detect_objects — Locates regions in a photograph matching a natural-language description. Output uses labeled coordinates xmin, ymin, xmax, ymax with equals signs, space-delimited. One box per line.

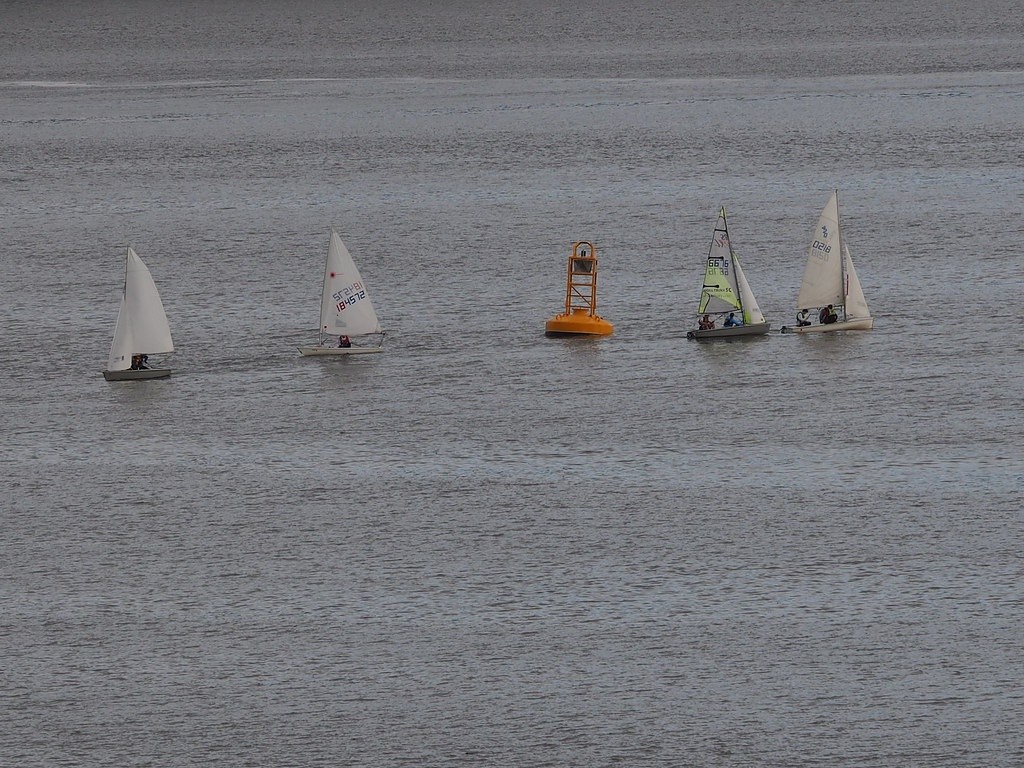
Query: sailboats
xmin=102 ymin=245 xmax=175 ymax=381
xmin=296 ymin=227 xmax=387 ymax=356
xmin=687 ymin=205 xmax=771 ymax=338
xmin=780 ymin=189 xmax=874 ymax=333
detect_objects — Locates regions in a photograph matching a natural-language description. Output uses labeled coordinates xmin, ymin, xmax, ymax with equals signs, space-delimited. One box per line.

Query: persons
xmin=796 ymin=309 xmax=811 ymax=327
xmin=339 ymin=335 xmax=351 ymax=348
xmin=132 ymin=354 xmax=149 ymax=370
xmin=824 ymin=304 xmax=838 ymax=324
xmin=724 ymin=313 xmax=740 ymax=327
xmin=698 ymin=316 xmax=714 ymax=330
